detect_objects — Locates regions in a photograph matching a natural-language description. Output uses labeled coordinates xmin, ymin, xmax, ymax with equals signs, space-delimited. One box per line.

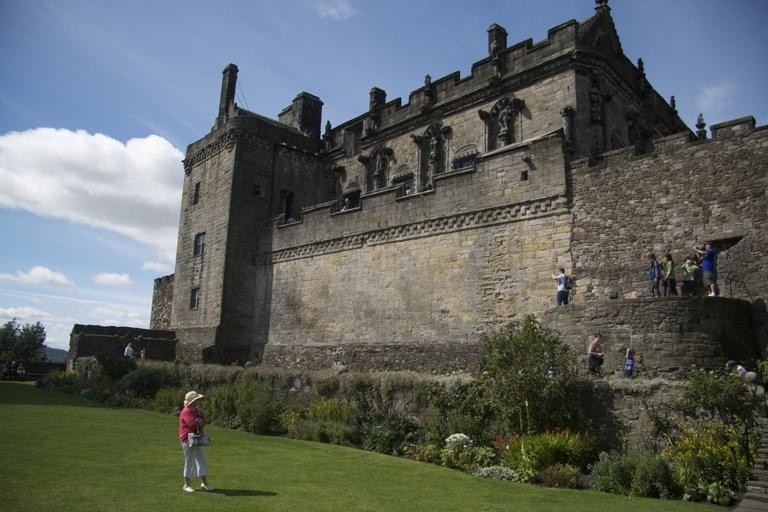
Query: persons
xmin=341 ymin=199 xmax=353 ymax=211
xmin=625 ymin=348 xmax=636 ymax=378
xmin=420 ymin=181 xmax=427 ymax=192
xmin=587 ymin=333 xmax=604 ymax=375
xmin=427 ymin=183 xmax=432 ymax=191
xmin=0 ymin=359 xmax=27 ymax=381
xmin=718 ymin=360 xmax=751 ymax=383
xmin=139 ymin=345 xmax=146 ymax=359
xmin=404 ymin=183 xmax=412 ymax=196
xmin=646 ymin=242 xmax=716 ymax=298
xmin=552 ymin=266 xmax=569 ymax=305
xmin=285 ymin=212 xmax=296 ymax=224
xmin=123 ymin=342 xmax=133 ymax=359
xmin=179 ymin=390 xmax=213 ymax=492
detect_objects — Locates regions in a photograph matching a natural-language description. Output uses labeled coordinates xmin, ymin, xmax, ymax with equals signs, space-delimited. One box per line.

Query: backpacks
xmin=563 ymin=273 xmax=573 ymax=290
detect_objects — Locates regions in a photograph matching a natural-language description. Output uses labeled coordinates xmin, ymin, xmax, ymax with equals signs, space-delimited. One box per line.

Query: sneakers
xmin=182 ymin=484 xmax=195 ymax=493
xmin=201 ymin=483 xmax=210 ymax=492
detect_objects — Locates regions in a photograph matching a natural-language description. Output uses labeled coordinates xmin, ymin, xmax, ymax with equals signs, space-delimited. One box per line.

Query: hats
xmin=184 ymin=390 xmax=204 ymax=408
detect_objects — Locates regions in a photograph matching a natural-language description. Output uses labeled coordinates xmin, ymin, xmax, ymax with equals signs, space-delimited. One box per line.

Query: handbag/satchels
xmin=188 ymin=433 xmax=210 ymax=448
